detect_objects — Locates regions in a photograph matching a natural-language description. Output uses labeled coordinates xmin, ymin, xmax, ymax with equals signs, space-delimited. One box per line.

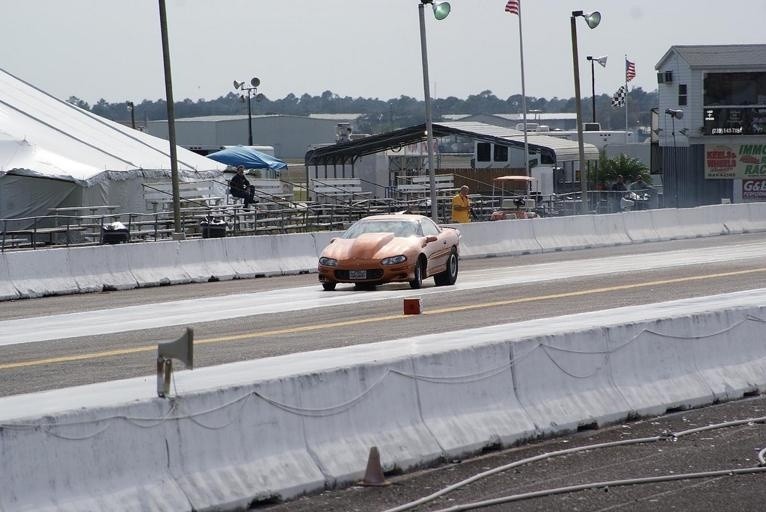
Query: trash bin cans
xmin=200 ymin=220 xmax=227 ymax=238
xmin=630 ymin=196 xmax=650 ymax=211
xmin=102 ymin=226 xmax=130 ymax=245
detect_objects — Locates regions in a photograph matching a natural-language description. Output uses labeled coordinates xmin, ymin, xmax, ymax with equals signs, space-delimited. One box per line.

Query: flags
xmin=505 ymin=0 xmax=519 ymax=14
xmin=626 ymin=60 xmax=635 ymax=82
xmin=611 ymin=85 xmax=628 ymax=107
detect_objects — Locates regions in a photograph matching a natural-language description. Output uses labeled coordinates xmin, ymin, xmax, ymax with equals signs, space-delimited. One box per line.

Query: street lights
xmin=234 ymin=76 xmax=265 ymax=145
xmin=569 ymin=8 xmax=603 ymax=212
xmin=586 ymin=55 xmax=613 ymax=124
xmin=125 ymin=101 xmax=137 ymax=128
xmin=416 ymin=0 xmax=453 ymax=223
xmin=664 ymin=107 xmax=685 ymax=205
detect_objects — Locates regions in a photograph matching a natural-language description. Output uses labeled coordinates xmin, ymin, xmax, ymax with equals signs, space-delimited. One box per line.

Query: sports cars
xmin=314 ymin=210 xmax=463 ymax=291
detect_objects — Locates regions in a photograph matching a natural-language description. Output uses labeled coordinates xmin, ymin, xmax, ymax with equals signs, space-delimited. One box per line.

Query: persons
xmin=230 ymin=165 xmax=259 ymax=212
xmin=630 ymin=175 xmax=652 ymax=190
xmin=612 ymin=175 xmax=628 ymax=190
xmin=451 ymin=185 xmax=472 ymax=223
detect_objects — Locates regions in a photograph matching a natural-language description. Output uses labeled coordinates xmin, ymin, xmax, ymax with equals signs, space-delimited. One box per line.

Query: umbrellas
xmin=206 ymin=144 xmax=288 ymax=171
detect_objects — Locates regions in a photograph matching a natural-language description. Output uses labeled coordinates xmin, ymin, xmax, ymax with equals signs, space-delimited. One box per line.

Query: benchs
xmin=0 ymin=171 xmax=542 ymax=250
